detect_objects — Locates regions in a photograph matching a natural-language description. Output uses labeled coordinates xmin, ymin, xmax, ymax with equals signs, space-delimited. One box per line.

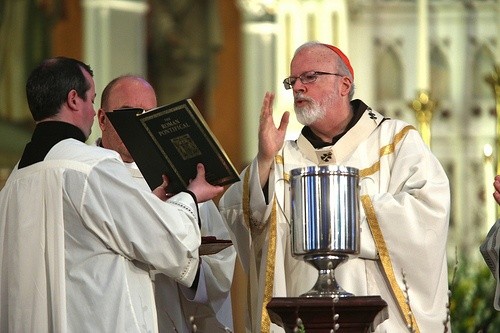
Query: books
xmin=199 ymin=237 xmax=232 ymax=255
xmin=105 ymin=99 xmax=241 ymax=198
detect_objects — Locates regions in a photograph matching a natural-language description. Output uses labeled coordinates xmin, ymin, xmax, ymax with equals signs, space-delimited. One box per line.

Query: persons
xmin=218 ymin=41 xmax=449 ymax=333
xmin=0 ymin=55 xmax=225 ymax=333
xmin=91 ymin=75 xmax=237 ymax=333
xmin=478 ymin=174 xmax=500 ymax=332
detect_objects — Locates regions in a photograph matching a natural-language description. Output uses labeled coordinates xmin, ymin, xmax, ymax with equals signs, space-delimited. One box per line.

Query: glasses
xmin=283 ymin=69 xmax=344 ymax=90
